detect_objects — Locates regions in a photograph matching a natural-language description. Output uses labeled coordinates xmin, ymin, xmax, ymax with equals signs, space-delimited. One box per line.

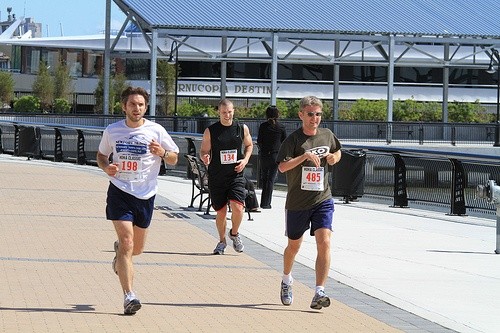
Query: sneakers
xmin=280 ymin=279 xmax=293 ymax=305
xmin=113 ymin=241 xmax=119 ymax=274
xmin=124 ymin=290 xmax=141 ymax=314
xmin=310 ymin=290 xmax=330 ymax=310
xmin=228 ymin=229 xmax=244 ymax=252
xmin=214 ymin=241 xmax=226 ymax=254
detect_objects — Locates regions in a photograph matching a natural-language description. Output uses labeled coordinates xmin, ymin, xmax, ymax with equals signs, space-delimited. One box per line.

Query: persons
xmin=200 ymin=99 xmax=253 ymax=255
xmin=276 ymin=96 xmax=342 ymax=310
xmin=258 ymin=105 xmax=287 ymax=209
xmin=98 ymin=86 xmax=180 ymax=315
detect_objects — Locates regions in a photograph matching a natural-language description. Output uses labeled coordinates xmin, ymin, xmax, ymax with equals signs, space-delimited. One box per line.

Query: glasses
xmin=303 ymin=112 xmax=322 ymax=117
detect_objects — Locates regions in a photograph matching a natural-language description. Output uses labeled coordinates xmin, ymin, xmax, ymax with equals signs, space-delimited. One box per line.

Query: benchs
xmin=183 ymin=154 xmax=254 ymax=220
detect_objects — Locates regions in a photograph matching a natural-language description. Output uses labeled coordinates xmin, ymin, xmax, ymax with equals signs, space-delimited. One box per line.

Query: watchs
xmin=159 ymin=149 xmax=168 ymax=159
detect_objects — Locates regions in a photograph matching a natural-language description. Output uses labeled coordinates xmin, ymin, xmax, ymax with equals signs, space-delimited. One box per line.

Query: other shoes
xmin=245 ymin=208 xmax=260 ymax=212
